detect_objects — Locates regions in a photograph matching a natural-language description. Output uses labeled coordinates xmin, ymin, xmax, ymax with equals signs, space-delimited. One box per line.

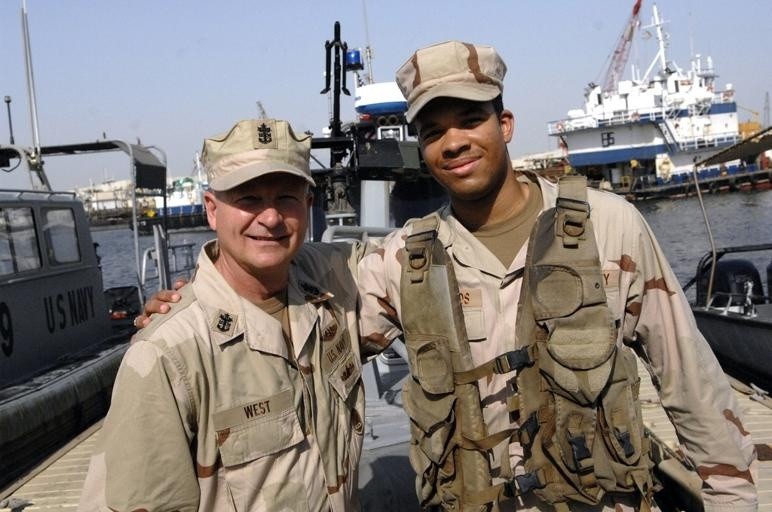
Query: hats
xmin=395 ymin=41 xmax=507 ymax=125
xmin=199 ymin=118 xmax=317 ymax=192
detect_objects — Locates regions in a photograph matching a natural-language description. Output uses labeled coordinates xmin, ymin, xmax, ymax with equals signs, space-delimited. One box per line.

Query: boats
xmin=0 ymin=2 xmax=195 ymax=471
xmin=1 ymin=178 xmax=134 ymax=238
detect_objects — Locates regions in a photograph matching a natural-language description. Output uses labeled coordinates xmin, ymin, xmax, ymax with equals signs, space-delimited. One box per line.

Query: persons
xmin=76 ymin=120 xmax=382 ymax=511
xmin=133 ymin=41 xmax=759 ymax=511
